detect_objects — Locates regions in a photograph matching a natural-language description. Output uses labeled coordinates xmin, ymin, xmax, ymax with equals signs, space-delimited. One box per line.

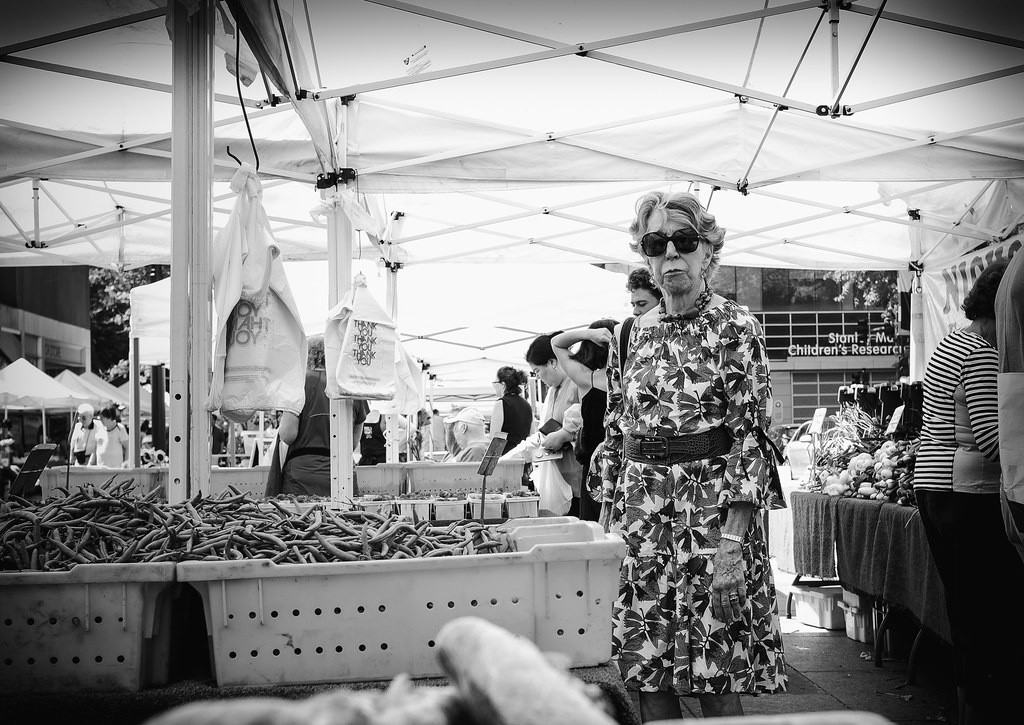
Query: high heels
xmin=956 ymin=675 xmax=999 ymax=724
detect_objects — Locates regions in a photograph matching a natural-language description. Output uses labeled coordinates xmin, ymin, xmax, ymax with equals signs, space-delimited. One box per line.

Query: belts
xmin=624 ymin=425 xmax=731 ymax=466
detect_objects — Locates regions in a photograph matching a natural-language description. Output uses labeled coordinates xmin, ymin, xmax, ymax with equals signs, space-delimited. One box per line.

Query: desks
xmin=786 ymin=492 xmax=951 ymax=689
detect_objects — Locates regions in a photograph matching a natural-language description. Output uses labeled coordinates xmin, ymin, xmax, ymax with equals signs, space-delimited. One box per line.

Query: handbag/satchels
xmin=264 ymin=433 xmax=282 ymax=497
xmin=74 ymin=450 xmax=86 ymax=465
xmin=997 ymin=372 xmax=1024 ymax=504
xmin=530 ymin=459 xmax=573 ymax=516
xmin=573 ymin=427 xmax=588 ymax=466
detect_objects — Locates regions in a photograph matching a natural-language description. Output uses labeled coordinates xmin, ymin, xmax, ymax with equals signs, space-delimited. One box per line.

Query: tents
xmin=129 ymin=266 xmax=633 ymax=468
xmin=2 ymin=0 xmax=1024 ymax=506
xmin=0 ymin=358 xmax=169 ymax=444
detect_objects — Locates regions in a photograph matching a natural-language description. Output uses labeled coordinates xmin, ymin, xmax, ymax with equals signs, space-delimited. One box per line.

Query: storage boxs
xmin=1 ymin=455 xmax=627 ymax=705
xmin=836 ymin=585 xmax=873 ymax=644
xmin=871 ymin=597 xmax=931 ymax=660
xmin=790 ymin=585 xmax=846 ymax=630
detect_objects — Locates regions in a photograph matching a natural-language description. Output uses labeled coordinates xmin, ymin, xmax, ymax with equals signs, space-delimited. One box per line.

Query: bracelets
xmin=721 ymin=533 xmax=744 ymax=551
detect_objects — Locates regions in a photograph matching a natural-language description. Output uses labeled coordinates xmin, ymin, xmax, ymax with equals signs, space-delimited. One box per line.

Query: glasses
xmin=640 ymin=227 xmax=710 ymax=257
xmin=492 ymin=381 xmax=503 ymax=387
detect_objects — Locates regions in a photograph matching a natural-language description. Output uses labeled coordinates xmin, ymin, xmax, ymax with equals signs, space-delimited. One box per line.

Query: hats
xmin=443 ymin=407 xmax=486 ymax=426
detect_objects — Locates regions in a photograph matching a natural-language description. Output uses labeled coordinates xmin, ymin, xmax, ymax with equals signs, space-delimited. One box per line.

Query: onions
xmin=818 ymin=440 xmax=897 ymax=501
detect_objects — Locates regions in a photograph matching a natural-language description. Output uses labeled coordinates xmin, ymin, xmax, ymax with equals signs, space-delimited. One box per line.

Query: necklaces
xmin=659 ymin=278 xmax=714 ymax=322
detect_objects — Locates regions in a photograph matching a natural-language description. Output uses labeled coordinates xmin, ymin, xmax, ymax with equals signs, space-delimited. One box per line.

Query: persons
xmin=212 ymin=414 xmax=225 ymax=454
xmin=433 ymin=409 xmax=445 ymax=451
xmin=913 ymin=244 xmax=1024 ymax=725
xmin=0 ymin=419 xmax=15 ymax=501
xmin=357 ymin=410 xmax=417 ymax=465
xmin=440 ymin=405 xmax=489 ymax=463
xmin=69 ymin=403 xmax=129 ymax=468
xmin=525 ymin=192 xmax=791 ymax=723
xmin=490 ymin=366 xmax=536 ymax=455
xmin=279 ymin=334 xmax=371 ymax=496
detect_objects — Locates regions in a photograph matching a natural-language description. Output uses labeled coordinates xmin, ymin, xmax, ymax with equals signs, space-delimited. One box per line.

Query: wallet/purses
xmin=539 ymin=419 xmax=571 ymax=449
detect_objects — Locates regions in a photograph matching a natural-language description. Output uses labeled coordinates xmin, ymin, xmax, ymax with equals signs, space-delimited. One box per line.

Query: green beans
xmin=0 ymin=473 xmax=522 ymax=575
